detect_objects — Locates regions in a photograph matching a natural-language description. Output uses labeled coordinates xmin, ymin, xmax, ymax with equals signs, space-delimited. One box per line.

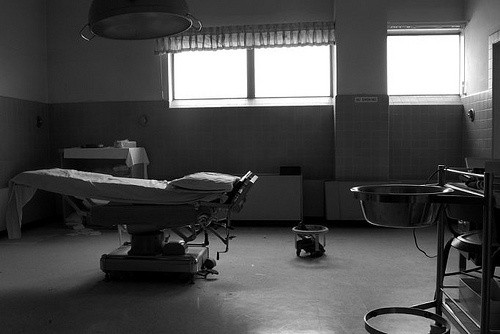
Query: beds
xmin=7 ymin=168 xmax=259 ymax=284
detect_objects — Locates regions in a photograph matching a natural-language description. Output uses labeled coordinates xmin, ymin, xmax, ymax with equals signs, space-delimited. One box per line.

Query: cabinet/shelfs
xmin=59 ymin=145 xmax=145 ymax=229
xmin=350 ymin=163 xmax=499 ymax=334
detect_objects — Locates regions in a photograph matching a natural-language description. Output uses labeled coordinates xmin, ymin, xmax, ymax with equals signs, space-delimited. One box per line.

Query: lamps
xmin=78 ymin=0 xmax=203 ymax=41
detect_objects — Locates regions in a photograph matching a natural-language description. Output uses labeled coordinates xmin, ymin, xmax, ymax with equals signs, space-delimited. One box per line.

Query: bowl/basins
xmin=350 ymin=182 xmax=454 ymax=228
